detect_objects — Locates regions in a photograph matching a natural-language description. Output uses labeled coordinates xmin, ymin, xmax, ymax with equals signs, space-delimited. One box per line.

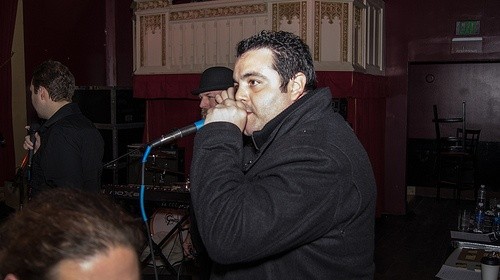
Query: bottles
xmin=492 ymin=204 xmax=500 ymax=237
xmin=474 ymin=203 xmax=485 ymax=233
xmin=477 ymin=185 xmax=486 ymax=203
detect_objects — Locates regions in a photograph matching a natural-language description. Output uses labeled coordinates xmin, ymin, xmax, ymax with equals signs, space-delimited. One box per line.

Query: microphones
xmin=28 ymin=123 xmax=40 ymax=182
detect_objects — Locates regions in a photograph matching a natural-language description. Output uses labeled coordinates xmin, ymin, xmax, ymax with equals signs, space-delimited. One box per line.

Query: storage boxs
xmin=73 ymin=84 xmax=145 ymax=189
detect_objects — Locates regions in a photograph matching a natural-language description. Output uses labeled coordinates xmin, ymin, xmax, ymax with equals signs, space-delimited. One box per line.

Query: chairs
xmin=430 ymin=102 xmax=481 ymax=206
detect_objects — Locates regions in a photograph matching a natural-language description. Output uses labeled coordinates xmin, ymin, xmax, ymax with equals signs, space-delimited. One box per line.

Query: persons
xmin=190 ymin=30 xmax=375 ymax=280
xmin=23 ymin=61 xmax=104 ymax=199
xmin=182 ymin=66 xmax=236 ymax=280
xmin=0 ymin=186 xmax=144 ymax=280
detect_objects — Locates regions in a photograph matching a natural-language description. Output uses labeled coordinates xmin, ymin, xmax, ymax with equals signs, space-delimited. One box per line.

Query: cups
xmin=479 ymin=256 xmax=500 ymax=280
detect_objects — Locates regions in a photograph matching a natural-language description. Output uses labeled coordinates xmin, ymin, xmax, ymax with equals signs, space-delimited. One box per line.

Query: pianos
xmin=102 ymin=183 xmax=195 ymax=280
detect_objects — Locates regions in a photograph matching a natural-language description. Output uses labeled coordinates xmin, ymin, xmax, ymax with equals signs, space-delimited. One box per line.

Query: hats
xmin=191 ymin=66 xmax=234 ymax=95
